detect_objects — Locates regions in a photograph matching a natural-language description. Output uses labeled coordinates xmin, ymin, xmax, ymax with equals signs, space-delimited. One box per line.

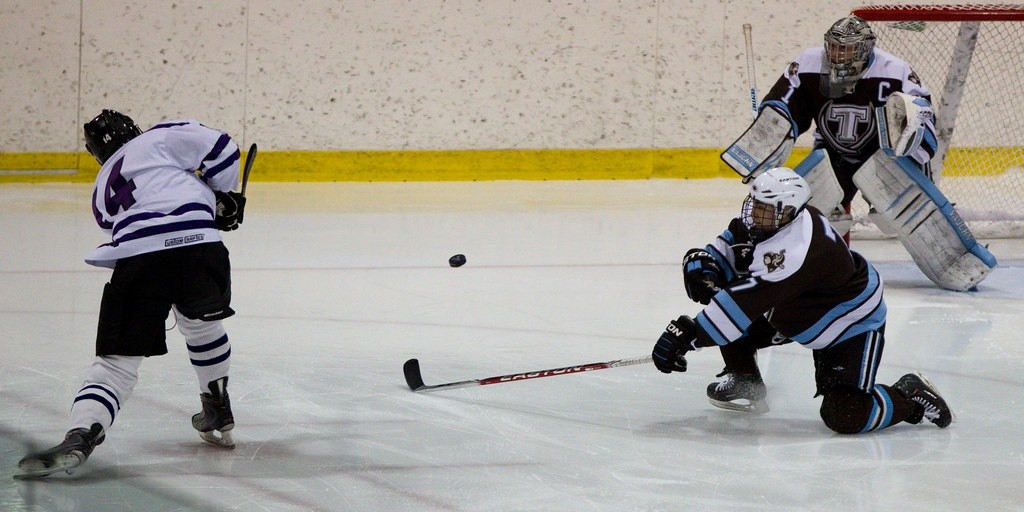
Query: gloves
xmin=684 ymin=249 xmax=723 ymax=304
xmin=651 ymin=316 xmax=697 ymax=374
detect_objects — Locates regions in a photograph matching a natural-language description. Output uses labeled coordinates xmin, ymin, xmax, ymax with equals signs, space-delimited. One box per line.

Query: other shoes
xmin=896 ymin=372 xmax=951 ymax=432
xmin=708 ymin=375 xmax=771 ymax=413
xmin=18 ymin=430 xmax=98 ymax=481
xmin=191 ymin=393 xmax=234 ymax=451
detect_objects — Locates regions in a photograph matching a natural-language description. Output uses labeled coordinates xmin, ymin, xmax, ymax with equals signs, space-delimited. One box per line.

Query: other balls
xmin=449 ymin=254 xmax=466 ymax=268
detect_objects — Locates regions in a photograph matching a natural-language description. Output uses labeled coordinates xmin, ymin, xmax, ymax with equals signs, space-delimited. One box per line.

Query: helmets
xmin=743 ymin=167 xmax=811 ymax=240
xmin=823 ymin=18 xmax=877 ymax=83
xmin=83 ymin=110 xmax=142 ymax=165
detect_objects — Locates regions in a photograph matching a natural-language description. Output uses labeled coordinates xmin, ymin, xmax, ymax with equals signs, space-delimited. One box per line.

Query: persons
xmin=12 ymin=109 xmax=240 ymax=477
xmin=757 ymin=16 xmax=997 ymax=291
xmin=651 ymin=166 xmax=957 ymax=433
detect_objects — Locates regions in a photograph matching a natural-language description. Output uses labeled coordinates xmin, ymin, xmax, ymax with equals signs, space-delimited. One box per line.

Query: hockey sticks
xmin=743 ymin=23 xmax=760 ymax=122
xmin=402 ymin=354 xmax=654 ymax=392
xmin=241 ymin=143 xmax=258 ymax=197
